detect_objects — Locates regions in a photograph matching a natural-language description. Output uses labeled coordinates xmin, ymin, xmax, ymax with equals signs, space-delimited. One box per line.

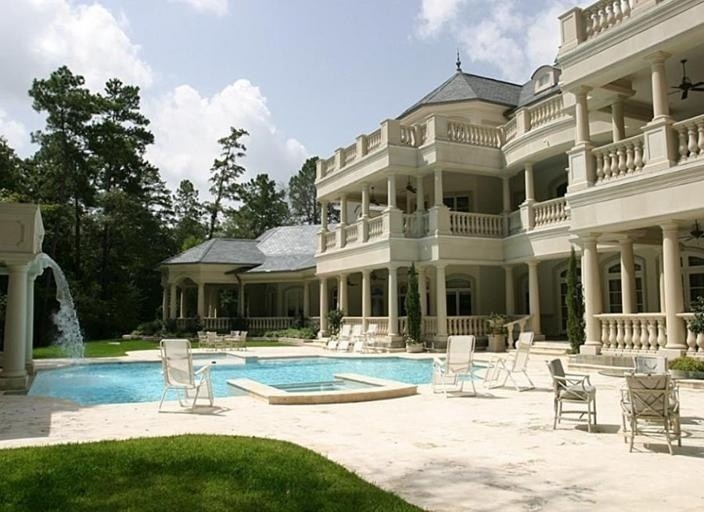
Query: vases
xmin=486 ymin=327 xmax=507 ymax=351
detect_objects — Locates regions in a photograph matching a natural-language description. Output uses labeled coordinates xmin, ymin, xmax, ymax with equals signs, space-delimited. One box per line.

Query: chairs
xmin=545 ymin=358 xmax=597 ymax=433
xmin=324 ymin=323 xmax=378 ymax=355
xmin=196 ymin=330 xmax=248 ymax=353
xmin=432 ymin=331 xmax=536 ymax=398
xmin=618 ymin=354 xmax=681 ymax=456
xmin=156 ymin=338 xmax=214 ymax=413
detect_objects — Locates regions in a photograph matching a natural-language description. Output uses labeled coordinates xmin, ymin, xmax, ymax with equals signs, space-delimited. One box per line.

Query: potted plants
xmin=403 ymin=261 xmax=426 ymax=352
xmin=666 ymin=355 xmax=704 ymax=379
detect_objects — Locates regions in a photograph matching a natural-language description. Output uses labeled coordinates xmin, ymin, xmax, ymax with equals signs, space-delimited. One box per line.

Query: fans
xmin=665 ymin=60 xmax=704 ymax=100
xmin=675 ymin=220 xmax=703 ymax=242
xmin=369 ymin=186 xmax=387 ymax=207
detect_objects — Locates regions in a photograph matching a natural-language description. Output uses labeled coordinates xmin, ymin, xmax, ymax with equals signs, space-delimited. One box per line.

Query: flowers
xmin=484 ymin=312 xmax=511 ymax=332
